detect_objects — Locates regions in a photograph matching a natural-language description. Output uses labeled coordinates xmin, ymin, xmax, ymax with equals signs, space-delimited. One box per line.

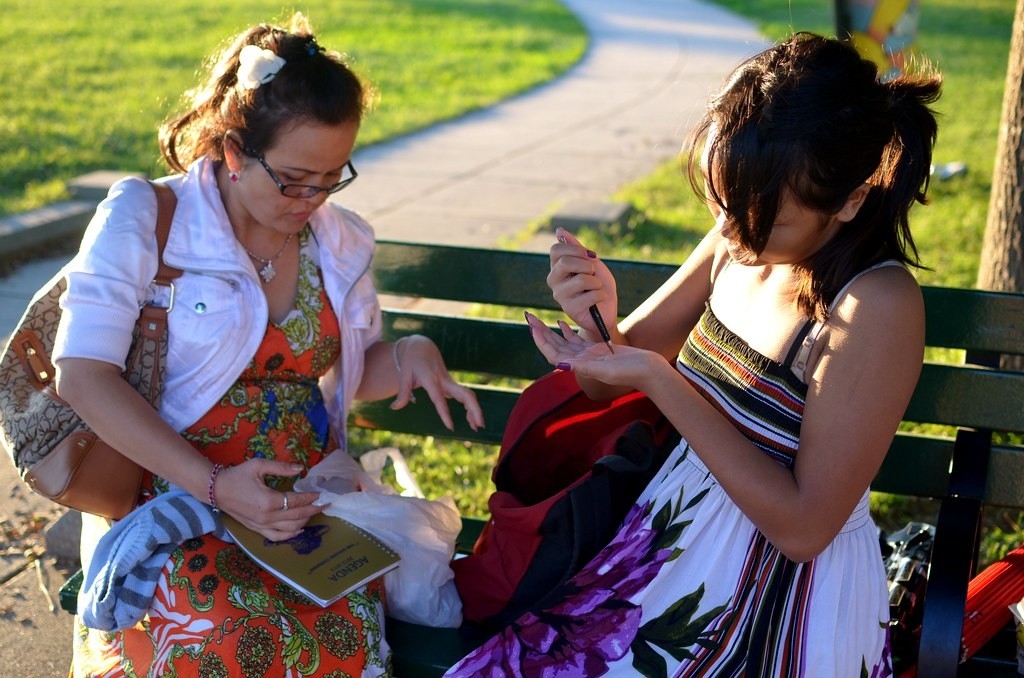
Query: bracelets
xmin=208 ymin=462 xmax=224 ymax=513
xmin=393 ymin=336 xmax=413 ymax=373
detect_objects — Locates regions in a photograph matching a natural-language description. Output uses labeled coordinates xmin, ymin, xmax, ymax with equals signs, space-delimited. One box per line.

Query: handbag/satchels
xmin=0 ymin=180 xmax=184 ymax=520
xmin=294 ymin=446 xmax=464 ymax=628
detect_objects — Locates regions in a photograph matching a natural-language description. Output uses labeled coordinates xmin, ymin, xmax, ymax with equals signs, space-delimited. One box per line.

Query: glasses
xmin=252 ymin=148 xmax=357 ymax=198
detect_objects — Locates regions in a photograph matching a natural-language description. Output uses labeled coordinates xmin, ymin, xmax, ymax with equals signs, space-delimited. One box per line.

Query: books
xmin=216 ymin=509 xmax=400 ymax=609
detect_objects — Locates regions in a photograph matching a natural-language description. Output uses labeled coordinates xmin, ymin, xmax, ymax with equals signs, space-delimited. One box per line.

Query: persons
xmin=440 ymin=27 xmax=943 ymax=678
xmin=48 ymin=10 xmax=488 ymax=678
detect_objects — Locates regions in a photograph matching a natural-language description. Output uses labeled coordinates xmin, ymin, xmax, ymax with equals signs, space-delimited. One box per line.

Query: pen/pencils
xmin=558 ymin=236 xmax=615 ymax=356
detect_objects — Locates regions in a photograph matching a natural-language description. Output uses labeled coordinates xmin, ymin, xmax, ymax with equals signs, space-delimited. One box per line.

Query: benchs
xmin=59 ymin=238 xmax=1024 ymax=678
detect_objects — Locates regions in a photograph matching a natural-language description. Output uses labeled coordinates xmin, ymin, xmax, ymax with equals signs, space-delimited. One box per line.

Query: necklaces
xmin=245 ymin=232 xmax=293 ymax=282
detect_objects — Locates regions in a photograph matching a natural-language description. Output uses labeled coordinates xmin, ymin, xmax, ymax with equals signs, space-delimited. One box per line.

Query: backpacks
xmin=450 ymin=369 xmax=683 ymax=630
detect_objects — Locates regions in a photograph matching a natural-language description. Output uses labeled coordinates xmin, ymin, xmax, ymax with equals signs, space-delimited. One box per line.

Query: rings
xmin=281 ymin=492 xmax=288 ymax=510
